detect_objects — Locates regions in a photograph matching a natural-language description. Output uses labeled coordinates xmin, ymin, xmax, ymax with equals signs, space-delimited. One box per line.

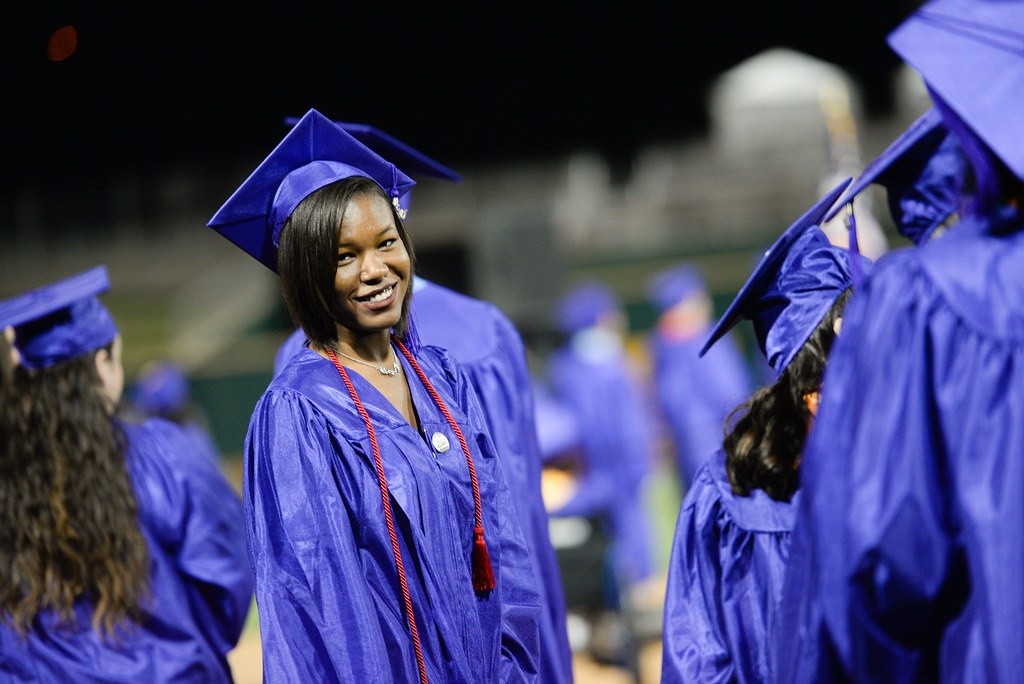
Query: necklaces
xmin=329 ymin=345 xmax=400 ymax=377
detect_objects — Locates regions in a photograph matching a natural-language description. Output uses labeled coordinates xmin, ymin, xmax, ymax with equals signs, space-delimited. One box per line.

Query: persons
xmin=0 ymin=0 xmax=1024 ymax=684
xmin=208 ymin=107 xmax=572 ymax=684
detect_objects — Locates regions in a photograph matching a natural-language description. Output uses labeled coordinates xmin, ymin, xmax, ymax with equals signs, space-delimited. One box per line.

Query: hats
xmin=649 ymin=264 xmax=698 ymax=316
xmin=887 ymin=0 xmax=1024 ymax=196
xmin=140 ymin=368 xmax=185 ymax=410
xmin=561 ymin=288 xmax=609 ymax=334
xmin=285 ymin=117 xmax=461 ymax=220
xmin=0 ymin=265 xmax=117 ymax=369
xmin=206 ymin=108 xmax=417 ymax=277
xmin=824 ymin=103 xmax=975 ymax=247
xmin=699 ymin=176 xmax=874 ymax=380
